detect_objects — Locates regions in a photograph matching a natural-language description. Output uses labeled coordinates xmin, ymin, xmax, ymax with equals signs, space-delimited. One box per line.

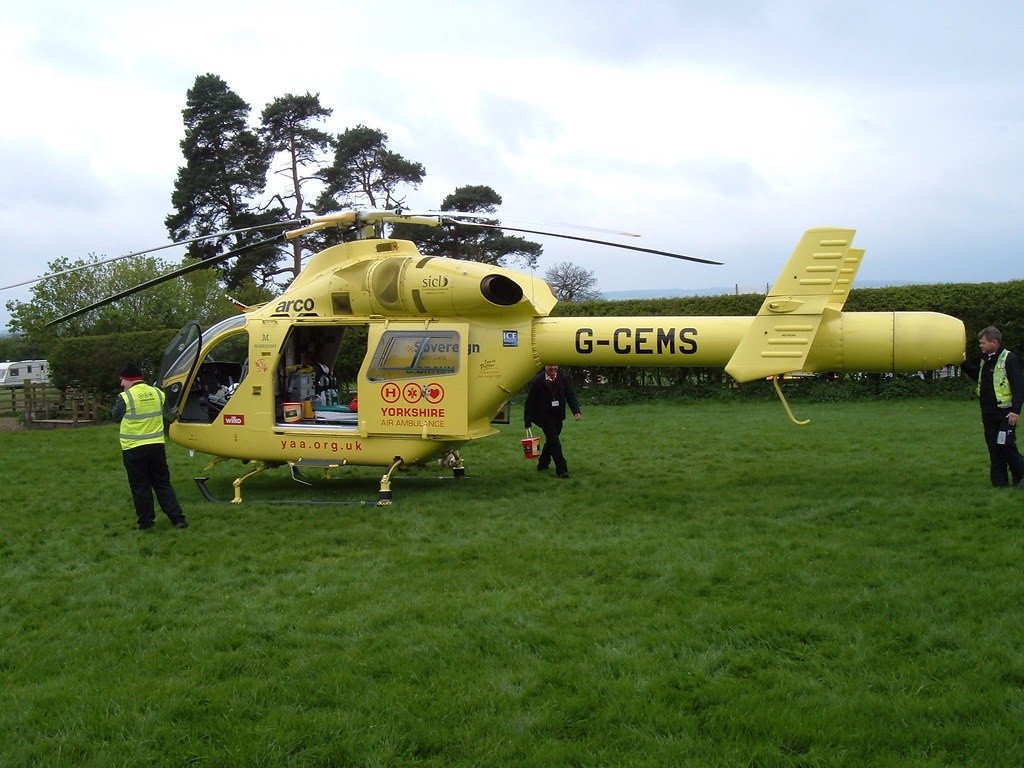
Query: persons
xmin=525 ymin=366 xmax=581 ymax=478
xmin=965 ymin=327 xmax=1023 ymax=488
xmin=116 ymin=364 xmax=190 ymax=528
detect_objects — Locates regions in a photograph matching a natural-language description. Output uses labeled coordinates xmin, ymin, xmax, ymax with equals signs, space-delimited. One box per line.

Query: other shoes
xmin=176 ymin=522 xmax=189 ymax=528
xmin=558 ymin=471 xmax=568 ymax=477
xmin=1012 ymin=476 xmax=1024 ymax=488
xmin=537 ymin=465 xmax=549 ymax=471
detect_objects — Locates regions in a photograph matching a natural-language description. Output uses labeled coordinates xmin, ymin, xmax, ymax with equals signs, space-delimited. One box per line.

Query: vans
xmin=0 ymin=359 xmax=52 ymax=388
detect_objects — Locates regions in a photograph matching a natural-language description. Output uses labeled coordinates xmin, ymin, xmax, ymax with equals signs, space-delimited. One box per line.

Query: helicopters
xmin=0 ymin=203 xmax=967 ymax=504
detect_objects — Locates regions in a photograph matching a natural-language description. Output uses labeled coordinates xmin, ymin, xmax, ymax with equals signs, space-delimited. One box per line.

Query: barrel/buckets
xmin=521 ymin=429 xmax=541 ymax=458
xmin=283 ymin=403 xmax=302 ymax=424
xmin=303 ymin=395 xmax=322 ymax=418
